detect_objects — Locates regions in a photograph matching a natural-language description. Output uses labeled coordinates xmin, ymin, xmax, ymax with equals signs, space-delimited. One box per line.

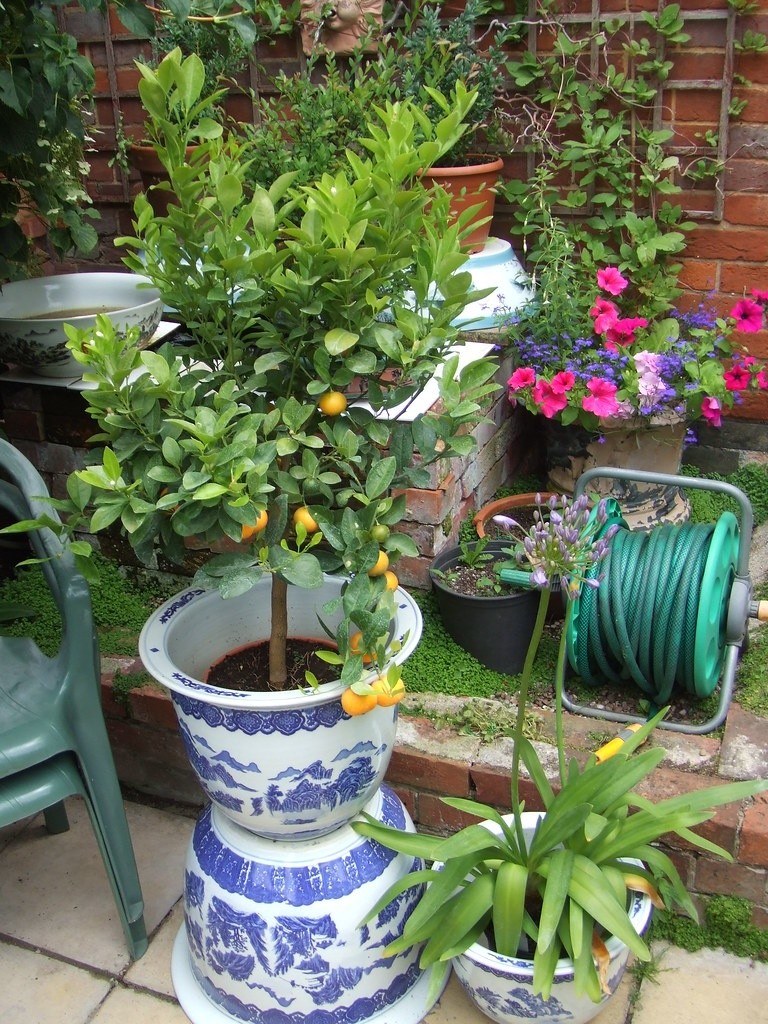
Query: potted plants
xmin=7 ymin=40 xmax=507 ymax=853
xmin=397 ymin=1 xmax=504 ymax=257
xmin=341 ymin=717 xmax=768 ymax=1024
xmin=429 ymin=539 xmax=542 ymax=677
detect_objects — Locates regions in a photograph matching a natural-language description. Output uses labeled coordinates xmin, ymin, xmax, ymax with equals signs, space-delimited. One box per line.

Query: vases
xmin=532 ymin=409 xmax=694 ymax=540
xmin=475 ymin=489 xmax=561 ymax=544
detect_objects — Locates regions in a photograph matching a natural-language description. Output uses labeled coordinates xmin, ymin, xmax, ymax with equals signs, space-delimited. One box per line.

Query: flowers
xmin=493 ymin=489 xmax=622 ymax=602
xmin=493 ymin=262 xmax=768 ymax=448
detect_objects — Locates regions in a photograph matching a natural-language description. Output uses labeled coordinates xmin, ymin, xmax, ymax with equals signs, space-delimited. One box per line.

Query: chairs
xmin=1 ymin=438 xmax=147 ymax=962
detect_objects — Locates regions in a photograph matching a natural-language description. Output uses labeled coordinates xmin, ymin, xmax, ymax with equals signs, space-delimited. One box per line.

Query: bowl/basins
xmin=372 ymin=237 xmax=542 ymax=332
xmin=0 ymin=272 xmax=164 ymax=378
